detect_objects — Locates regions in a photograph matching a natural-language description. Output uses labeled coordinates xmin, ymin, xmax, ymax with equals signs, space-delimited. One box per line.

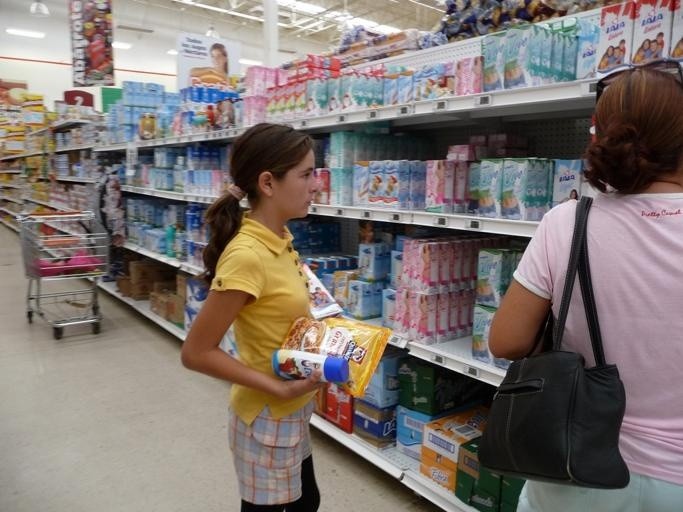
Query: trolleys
xmin=16 ymin=209 xmax=111 ymax=339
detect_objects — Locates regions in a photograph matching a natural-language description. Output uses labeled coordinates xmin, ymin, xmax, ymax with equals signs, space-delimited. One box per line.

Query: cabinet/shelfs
xmin=0 ymin=6 xmax=683 ymax=511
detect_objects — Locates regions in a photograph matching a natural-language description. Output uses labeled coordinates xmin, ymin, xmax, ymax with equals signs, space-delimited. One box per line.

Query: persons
xmin=489 ymin=65 xmax=683 ymax=512
xmin=210 ymin=43 xmax=229 ymax=77
xmin=181 ymin=123 xmax=322 ymax=512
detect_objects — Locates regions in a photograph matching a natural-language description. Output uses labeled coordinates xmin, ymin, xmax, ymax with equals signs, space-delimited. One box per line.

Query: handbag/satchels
xmin=476 ymin=195 xmax=630 ymax=489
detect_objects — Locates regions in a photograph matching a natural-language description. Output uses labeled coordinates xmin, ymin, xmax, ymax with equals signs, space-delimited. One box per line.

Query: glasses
xmin=595 ymin=59 xmax=683 ymax=104
